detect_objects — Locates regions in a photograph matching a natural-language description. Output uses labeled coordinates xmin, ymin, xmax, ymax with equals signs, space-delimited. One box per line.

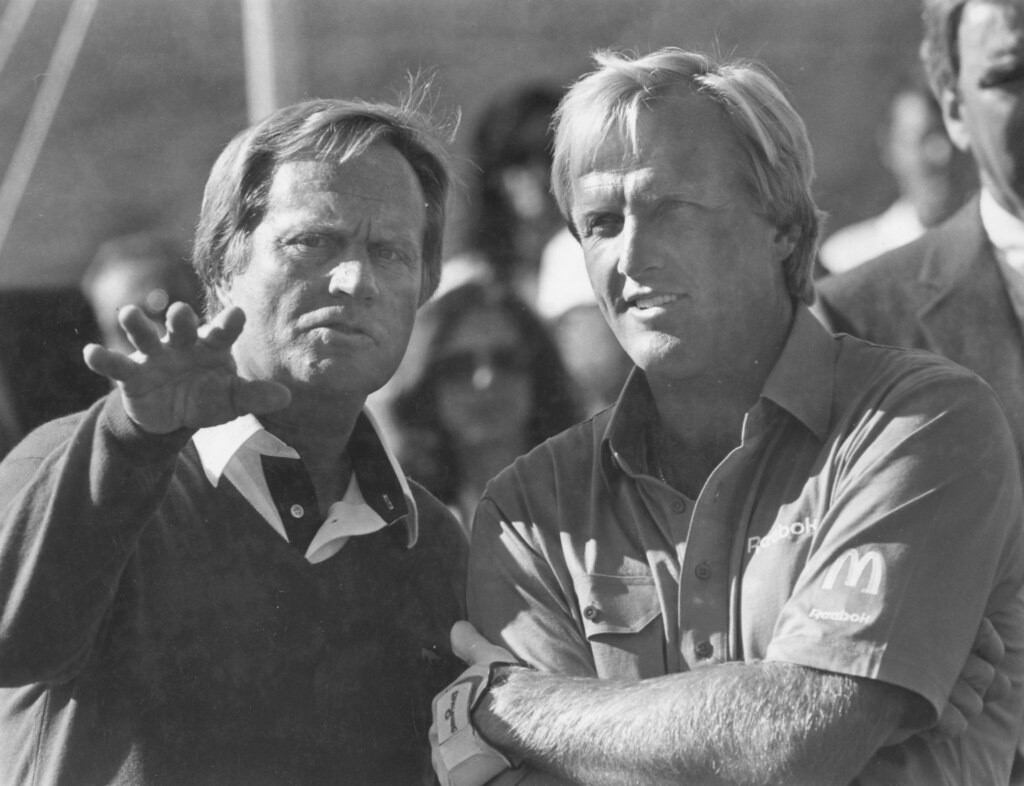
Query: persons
xmin=0 ymin=97 xmax=470 ymax=786
xmin=426 ymin=47 xmax=1024 ymax=786
xmin=809 ymin=1 xmax=1024 ymax=443
xmin=77 ymin=228 xmax=206 ymax=357
xmin=818 ymin=89 xmax=973 ymax=272
xmin=362 ymin=86 xmax=634 ymax=534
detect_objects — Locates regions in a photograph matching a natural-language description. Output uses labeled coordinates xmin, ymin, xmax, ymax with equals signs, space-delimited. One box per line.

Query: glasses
xmin=425 ymin=346 xmax=535 ymax=382
